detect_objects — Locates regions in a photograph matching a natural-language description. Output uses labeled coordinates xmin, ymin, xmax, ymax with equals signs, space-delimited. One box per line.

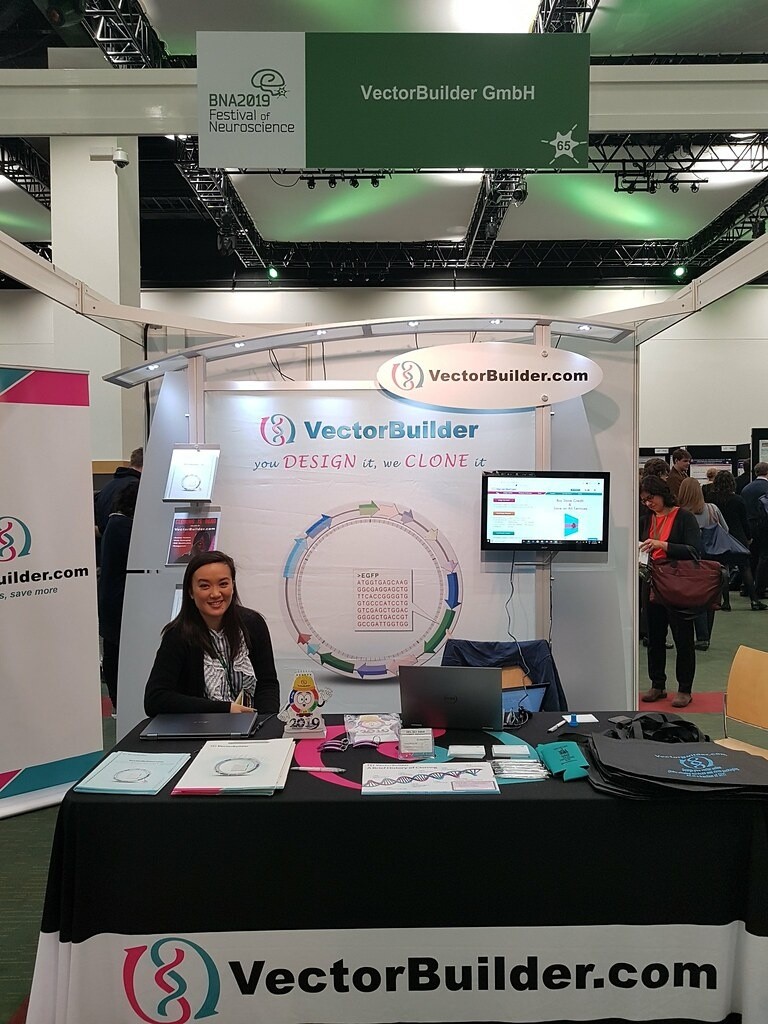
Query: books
xmin=491 ymin=744 xmax=530 ymax=758
xmin=170 ymin=738 xmax=296 ymax=796
xmin=74 ymin=751 xmax=191 ymax=795
xmin=360 ymin=761 xmax=500 ymax=795
xmin=447 ymin=745 xmax=485 ymax=759
xmin=399 ymin=728 xmax=435 ymax=758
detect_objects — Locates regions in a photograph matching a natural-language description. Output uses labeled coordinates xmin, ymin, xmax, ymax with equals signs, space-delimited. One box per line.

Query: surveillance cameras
xmin=112 ymin=149 xmax=129 ymax=169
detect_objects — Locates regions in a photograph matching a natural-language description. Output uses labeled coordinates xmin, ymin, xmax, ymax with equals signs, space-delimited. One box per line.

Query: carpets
xmin=638 ymin=691 xmax=725 ymax=715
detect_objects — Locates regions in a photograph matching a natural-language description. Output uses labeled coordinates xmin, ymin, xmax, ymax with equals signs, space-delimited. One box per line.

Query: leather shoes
xmin=641 ymin=686 xmax=667 ymax=703
xmin=671 ymin=690 xmax=692 ymax=708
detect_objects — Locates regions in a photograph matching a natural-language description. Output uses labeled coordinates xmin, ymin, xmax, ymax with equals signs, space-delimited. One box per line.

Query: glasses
xmin=664 ymin=471 xmax=671 ymax=480
xmin=641 ymin=494 xmax=655 ymax=506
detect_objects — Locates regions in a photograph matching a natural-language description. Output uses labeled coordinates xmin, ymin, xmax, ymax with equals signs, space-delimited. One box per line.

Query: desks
xmin=30 ymin=709 xmax=768 ymax=1024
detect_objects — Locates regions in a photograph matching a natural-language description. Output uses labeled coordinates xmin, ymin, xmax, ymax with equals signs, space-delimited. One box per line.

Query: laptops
xmin=139 ymin=711 xmax=258 ymax=740
xmin=399 ymin=665 xmax=505 ymax=731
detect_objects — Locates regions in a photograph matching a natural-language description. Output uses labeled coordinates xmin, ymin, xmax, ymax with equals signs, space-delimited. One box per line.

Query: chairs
xmin=439 ymin=638 xmax=571 ymax=713
xmin=713 ymin=644 xmax=768 ymax=760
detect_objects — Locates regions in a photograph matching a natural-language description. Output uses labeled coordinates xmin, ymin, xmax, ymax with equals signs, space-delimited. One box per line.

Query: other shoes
xmin=721 ymin=603 xmax=731 ymax=612
xmin=695 ymin=640 xmax=711 ymax=651
xmin=665 ymin=642 xmax=675 ymax=649
xmin=751 ymin=601 xmax=768 ymax=611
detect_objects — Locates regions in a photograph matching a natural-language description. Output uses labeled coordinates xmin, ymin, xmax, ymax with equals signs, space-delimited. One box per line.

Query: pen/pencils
xmin=547 ymin=719 xmax=567 ymax=733
xmin=290 ymin=766 xmax=347 ymax=773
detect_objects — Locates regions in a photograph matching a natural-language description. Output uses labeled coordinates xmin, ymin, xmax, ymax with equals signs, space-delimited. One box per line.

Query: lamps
xmin=751 ymin=219 xmax=766 ymax=238
xmin=486 ymin=222 xmax=497 ymax=241
xmin=671 ymin=264 xmax=688 ymax=280
xmin=621 ymin=179 xmax=709 ymax=195
xmin=327 ymin=270 xmax=391 ymax=282
xmin=512 ymin=182 xmax=529 ymax=208
xmin=297 ymin=173 xmax=386 ymax=190
xmin=216 ymin=212 xmax=237 ymax=259
xmin=44 ymin=5 xmax=65 ymax=26
xmin=267 ymin=264 xmax=281 ymax=279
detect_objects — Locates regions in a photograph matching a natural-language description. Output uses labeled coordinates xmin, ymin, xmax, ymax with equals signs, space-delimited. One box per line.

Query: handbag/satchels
xmin=646 ymin=546 xmax=724 ymax=611
xmin=700 ymin=503 xmax=753 ymax=561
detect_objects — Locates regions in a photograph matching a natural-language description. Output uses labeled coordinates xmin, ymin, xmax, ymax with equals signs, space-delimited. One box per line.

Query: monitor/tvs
xmin=481 ymin=470 xmax=610 ymax=552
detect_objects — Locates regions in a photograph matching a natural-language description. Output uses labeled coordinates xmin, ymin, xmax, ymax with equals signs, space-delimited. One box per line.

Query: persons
xmin=93 ymin=448 xmax=143 ymax=708
xmin=144 ymin=552 xmax=281 ymax=717
xmin=640 ymin=450 xmax=768 ymax=707
